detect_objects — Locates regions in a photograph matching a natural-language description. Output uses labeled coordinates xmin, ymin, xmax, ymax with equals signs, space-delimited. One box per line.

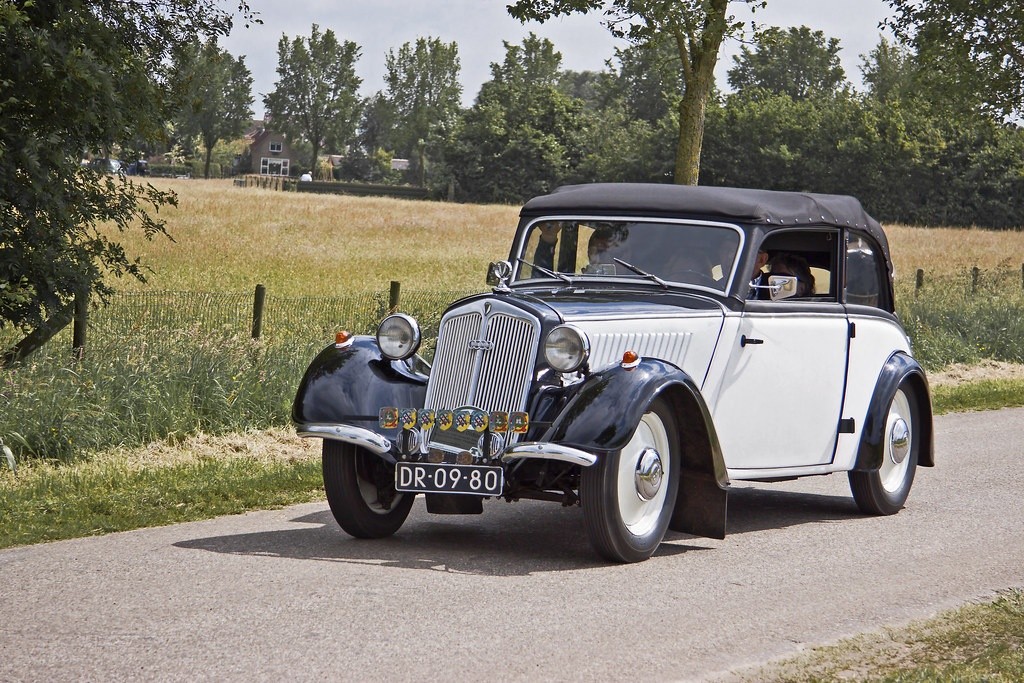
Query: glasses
xmin=768 ymin=272 xmax=804 ymax=283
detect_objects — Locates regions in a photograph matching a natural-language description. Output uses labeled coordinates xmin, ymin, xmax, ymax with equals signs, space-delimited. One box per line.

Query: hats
xmin=723 ymin=228 xmax=767 ymax=253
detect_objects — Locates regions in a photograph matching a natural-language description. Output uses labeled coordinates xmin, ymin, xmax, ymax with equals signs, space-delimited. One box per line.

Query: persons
xmin=656 ymin=233 xmax=818 ymax=301
xmin=530 ymin=221 xmax=636 ymax=276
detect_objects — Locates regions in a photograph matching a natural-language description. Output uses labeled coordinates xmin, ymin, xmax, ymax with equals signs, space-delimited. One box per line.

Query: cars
xmin=85 ymin=157 xmax=123 ymax=175
xmin=290 ymin=182 xmax=936 ymax=565
